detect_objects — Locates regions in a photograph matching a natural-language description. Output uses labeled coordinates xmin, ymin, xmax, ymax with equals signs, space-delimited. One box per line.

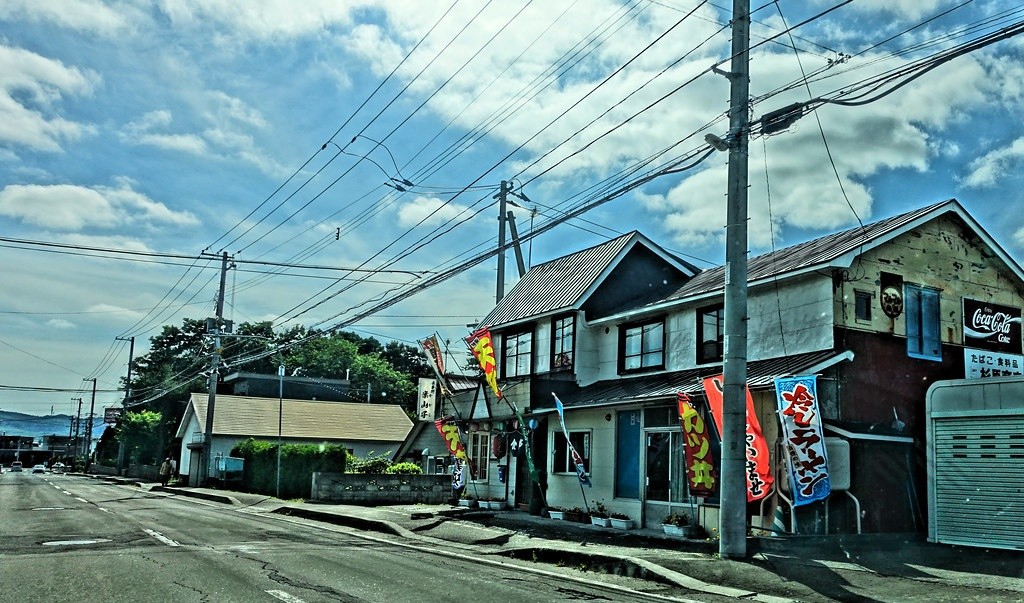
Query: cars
xmin=11 ymin=461 xmax=23 ymax=472
xmin=32 ymin=465 xmax=46 ymax=474
xmin=51 ymin=462 xmax=65 ymax=473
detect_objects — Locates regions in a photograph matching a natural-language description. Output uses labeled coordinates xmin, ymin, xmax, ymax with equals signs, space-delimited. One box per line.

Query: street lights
xmin=201 ymin=333 xmax=284 ymax=498
xmin=83 ymin=413 xmax=97 ymax=454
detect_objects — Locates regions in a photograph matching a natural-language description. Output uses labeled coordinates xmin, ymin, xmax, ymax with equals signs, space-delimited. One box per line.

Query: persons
xmin=160 ymin=457 xmax=172 ymax=486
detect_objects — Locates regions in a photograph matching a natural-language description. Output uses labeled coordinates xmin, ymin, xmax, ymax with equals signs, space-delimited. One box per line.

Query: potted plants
xmin=609 ymin=513 xmax=634 ymax=530
xmin=548 ymin=506 xmax=566 ymax=520
xmin=458 ymin=494 xmax=475 ymax=506
xmin=589 ymin=498 xmax=610 ymax=528
xmin=489 ymin=497 xmax=508 ymax=510
xmin=661 ymin=510 xmax=692 ymax=537
xmin=478 ymin=498 xmax=489 ymax=509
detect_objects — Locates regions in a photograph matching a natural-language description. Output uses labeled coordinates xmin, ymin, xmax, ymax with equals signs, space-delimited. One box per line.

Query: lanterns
xmin=493 ymin=434 xmax=506 ymax=463
